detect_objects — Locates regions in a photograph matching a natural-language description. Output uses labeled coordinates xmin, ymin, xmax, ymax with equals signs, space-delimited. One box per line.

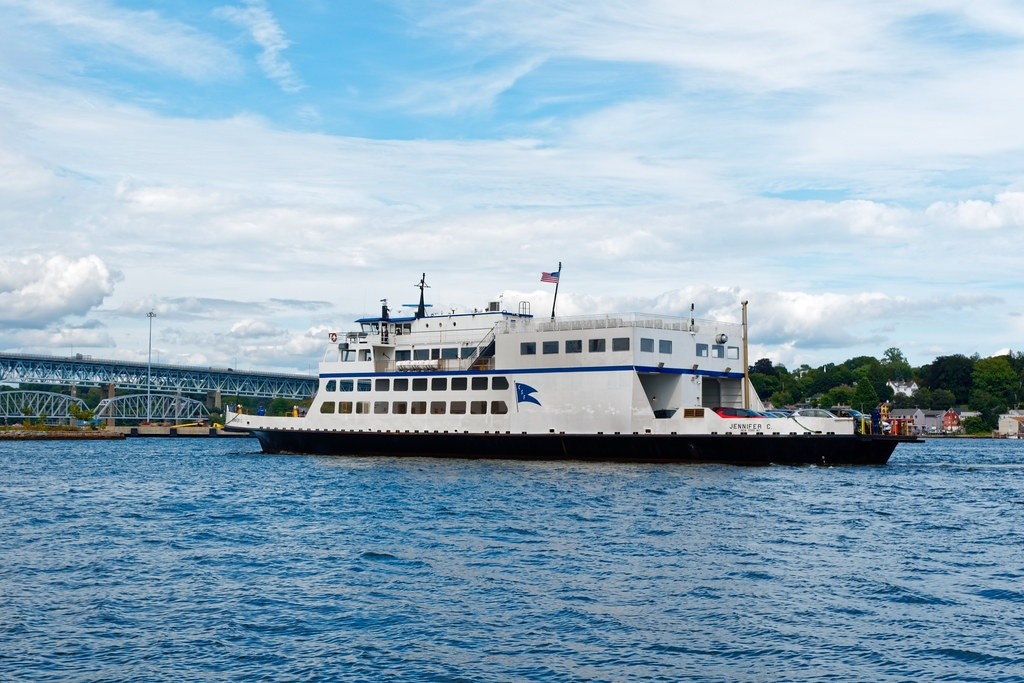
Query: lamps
xmin=725 ymin=368 xmax=731 ymax=372
xmin=659 ymin=363 xmax=666 ymax=367
xmin=653 ymin=397 xmax=655 ymax=400
xmin=693 ymin=365 xmax=698 ymax=370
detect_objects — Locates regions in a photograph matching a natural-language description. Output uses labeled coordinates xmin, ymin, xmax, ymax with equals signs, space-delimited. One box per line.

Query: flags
xmin=540 ymin=271 xmax=559 ymax=283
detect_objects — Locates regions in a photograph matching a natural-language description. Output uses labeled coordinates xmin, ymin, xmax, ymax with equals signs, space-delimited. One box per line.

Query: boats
xmin=219 ymin=258 xmax=930 ymax=467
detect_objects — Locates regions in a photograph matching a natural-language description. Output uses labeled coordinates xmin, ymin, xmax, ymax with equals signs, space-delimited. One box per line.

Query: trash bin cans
xmin=891 ymin=421 xmax=899 ymax=435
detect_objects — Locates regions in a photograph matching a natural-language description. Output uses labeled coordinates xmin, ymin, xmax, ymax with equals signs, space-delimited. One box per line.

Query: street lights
xmin=145 ymin=312 xmax=158 ymax=423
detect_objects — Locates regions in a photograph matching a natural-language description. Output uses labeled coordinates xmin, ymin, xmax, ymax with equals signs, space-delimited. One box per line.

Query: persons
xmin=871 ymin=409 xmax=881 ymax=435
xmin=384 ymin=327 xmax=388 ymax=339
